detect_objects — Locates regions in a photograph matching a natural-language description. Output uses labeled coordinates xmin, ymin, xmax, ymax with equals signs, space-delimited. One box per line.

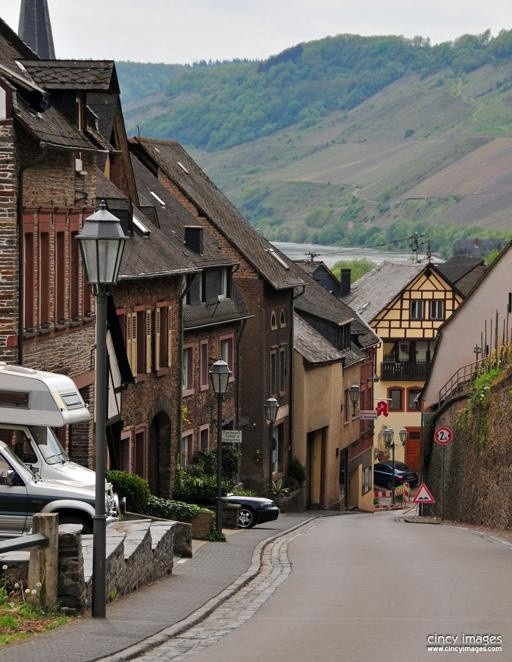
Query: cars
xmin=220 ymin=494 xmax=281 ymax=530
xmin=374 ymin=460 xmax=420 ymax=493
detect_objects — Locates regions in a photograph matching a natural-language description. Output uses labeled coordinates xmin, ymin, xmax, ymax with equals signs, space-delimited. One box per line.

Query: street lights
xmin=264 ymin=397 xmax=279 ymax=496
xmin=208 ymin=354 xmax=232 ymax=534
xmin=69 ymin=199 xmax=132 ymax=618
xmin=383 ymin=426 xmax=409 ymax=505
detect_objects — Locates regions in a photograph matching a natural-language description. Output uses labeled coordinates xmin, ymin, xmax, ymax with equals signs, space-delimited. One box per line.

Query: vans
xmin=0 ymin=362 xmax=121 ymax=543
